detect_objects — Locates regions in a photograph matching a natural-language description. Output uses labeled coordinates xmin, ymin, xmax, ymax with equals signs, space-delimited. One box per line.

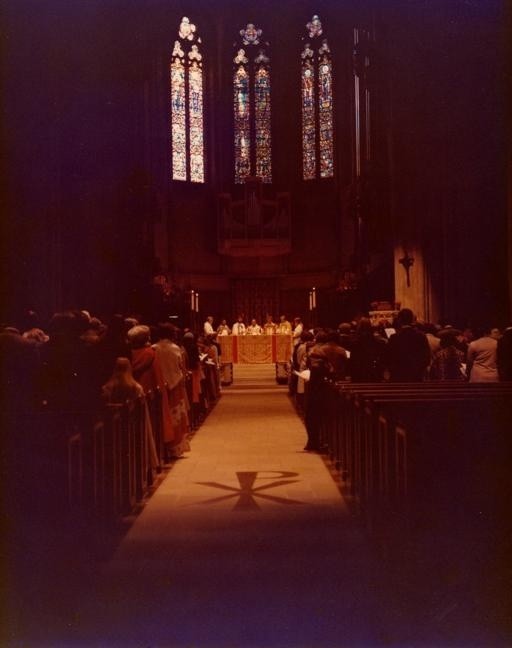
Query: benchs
xmin=282 ymin=357 xmax=512 ymax=617
xmin=2 ymin=359 xmax=218 ymax=627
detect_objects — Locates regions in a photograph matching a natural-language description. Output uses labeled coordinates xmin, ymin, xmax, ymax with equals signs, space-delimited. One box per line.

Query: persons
xmin=1 ymin=308 xmax=221 ymax=403
xmin=203 ymin=313 xmax=303 ymax=344
xmin=292 ymin=309 xmax=511 ymax=383
